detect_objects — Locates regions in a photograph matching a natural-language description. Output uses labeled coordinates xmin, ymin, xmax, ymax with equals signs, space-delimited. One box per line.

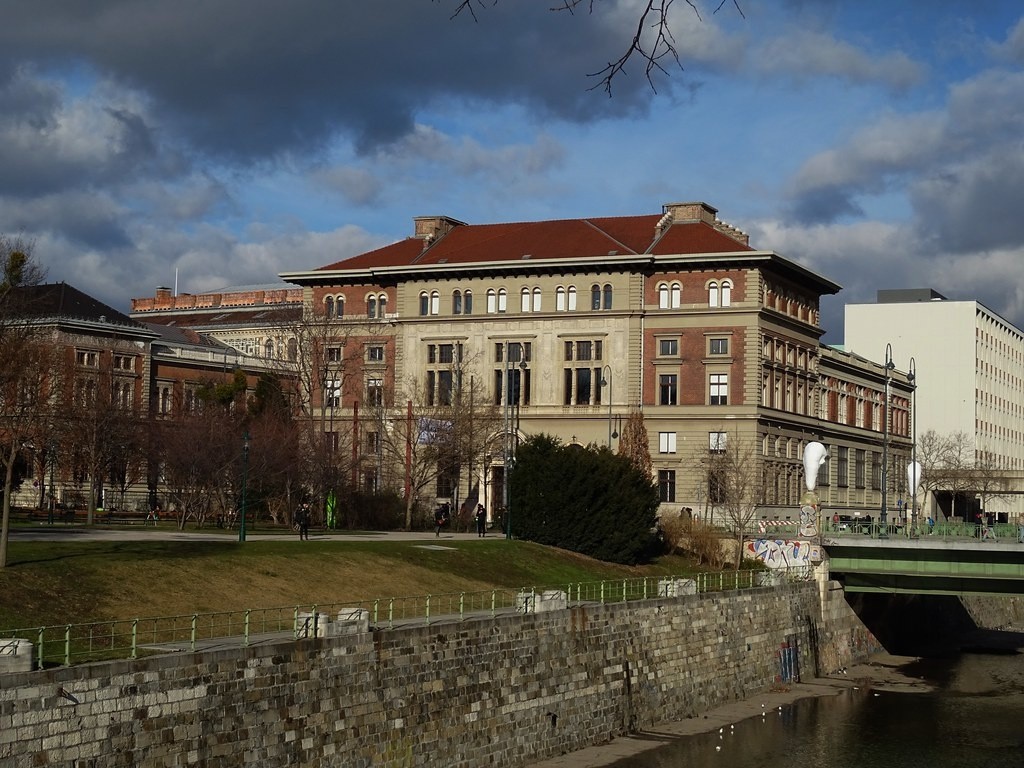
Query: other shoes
xmin=306 ymin=537 xmax=308 ymax=540
xmin=482 ymin=535 xmax=484 ymax=537
xmin=478 ymin=535 xmax=481 ymax=537
xmin=300 ymin=538 xmax=302 ymax=541
xmin=928 ymin=532 xmax=934 ymax=535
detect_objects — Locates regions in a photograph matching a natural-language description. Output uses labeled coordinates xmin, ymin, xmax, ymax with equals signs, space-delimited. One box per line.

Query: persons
xmin=865 ymin=512 xmax=871 ymax=534
xmin=435 ymin=502 xmax=449 ymax=537
xmin=294 ymin=503 xmax=310 ymax=540
xmin=973 ymin=512 xmax=1000 ymax=542
xmin=147 ymin=506 xmax=159 ymax=519
xmin=499 ymin=506 xmax=508 ymax=534
xmin=476 ymin=504 xmax=486 ymax=537
xmin=679 ymin=507 xmax=692 ymax=531
xmin=927 ymin=517 xmax=935 ymax=535
xmin=833 ymin=512 xmax=846 ymax=532
xmin=1019 ymin=513 xmax=1024 ymax=543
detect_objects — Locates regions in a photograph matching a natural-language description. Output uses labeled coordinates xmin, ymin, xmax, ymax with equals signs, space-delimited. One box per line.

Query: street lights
xmin=879 ymin=343 xmax=891 ymax=538
xmin=239 ymin=430 xmax=249 ymax=542
xmin=601 ymin=364 xmax=611 ymax=450
xmin=505 ymin=341 xmax=528 ymax=538
xmin=906 ymin=357 xmax=919 ymax=539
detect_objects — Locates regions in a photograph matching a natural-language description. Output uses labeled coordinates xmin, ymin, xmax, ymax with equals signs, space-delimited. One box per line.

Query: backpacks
xmin=932 ymin=520 xmax=935 ymax=526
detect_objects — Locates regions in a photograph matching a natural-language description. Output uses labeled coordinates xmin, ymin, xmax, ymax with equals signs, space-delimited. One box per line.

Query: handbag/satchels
xmin=474 ymin=516 xmax=479 ymax=522
